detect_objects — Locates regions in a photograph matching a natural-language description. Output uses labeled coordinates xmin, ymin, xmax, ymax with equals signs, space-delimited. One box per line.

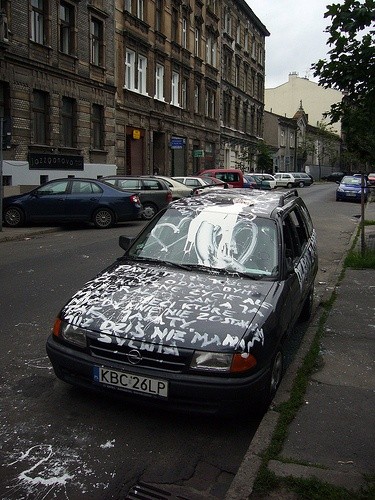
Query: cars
xmin=201 ymin=176 xmax=234 ymax=188
xmin=242 ymin=175 xmax=272 ymax=190
xmin=120 ymin=175 xmax=193 ymax=201
xmin=170 ymin=177 xmax=224 ymax=196
xmin=354 ymin=174 xmax=370 ymax=186
xmin=368 ymin=173 xmax=375 ymax=185
xmin=322 ymin=172 xmax=344 ymax=182
xmin=335 ymin=176 xmax=372 ymax=204
xmin=0 ymin=177 xmax=144 ymax=229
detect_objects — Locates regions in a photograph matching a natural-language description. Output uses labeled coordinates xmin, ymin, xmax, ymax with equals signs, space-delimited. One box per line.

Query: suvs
xmin=246 ymin=174 xmax=278 ymax=189
xmin=272 ymin=172 xmax=313 ymax=189
xmin=80 ymin=174 xmax=173 ymax=220
xmin=45 ymin=182 xmax=319 ymax=417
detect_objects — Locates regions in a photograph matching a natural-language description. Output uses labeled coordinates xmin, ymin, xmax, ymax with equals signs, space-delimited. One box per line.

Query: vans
xmin=198 ymin=169 xmax=244 ymax=188
